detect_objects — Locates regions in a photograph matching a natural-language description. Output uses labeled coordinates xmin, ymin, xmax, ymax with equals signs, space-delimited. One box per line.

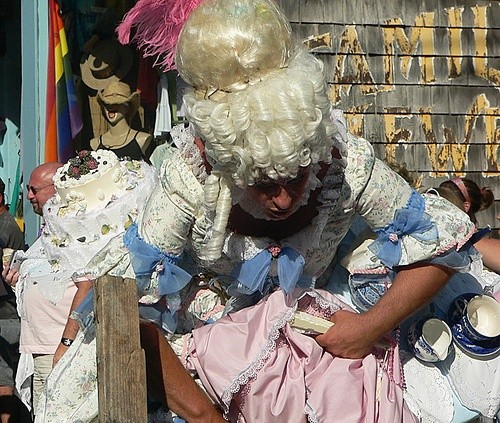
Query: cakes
xmin=42 ymin=147 xmax=161 ymax=271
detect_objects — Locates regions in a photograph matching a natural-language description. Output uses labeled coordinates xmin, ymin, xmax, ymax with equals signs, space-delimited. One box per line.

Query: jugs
xmin=407 ymin=313 xmax=454 ymax=363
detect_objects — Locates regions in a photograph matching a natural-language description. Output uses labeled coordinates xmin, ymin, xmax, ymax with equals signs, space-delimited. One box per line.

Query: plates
xmin=446 ymin=292 xmax=500 ymax=357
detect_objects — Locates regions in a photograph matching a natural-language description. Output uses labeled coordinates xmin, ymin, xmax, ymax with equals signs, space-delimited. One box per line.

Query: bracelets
xmin=61 ymin=337 xmax=74 ymax=346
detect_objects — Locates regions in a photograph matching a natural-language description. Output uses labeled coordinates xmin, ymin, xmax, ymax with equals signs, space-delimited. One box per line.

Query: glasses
xmin=26 ymin=184 xmax=53 ymax=195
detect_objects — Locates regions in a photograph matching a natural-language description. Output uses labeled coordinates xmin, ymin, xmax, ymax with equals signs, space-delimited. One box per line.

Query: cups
xmin=455 ymin=294 xmax=500 ymax=344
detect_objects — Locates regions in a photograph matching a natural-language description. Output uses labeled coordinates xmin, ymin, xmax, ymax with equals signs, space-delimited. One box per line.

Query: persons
xmin=107 ymin=0 xmax=475 ymax=423
xmin=0 ymin=162 xmax=94 ymax=423
xmin=421 ymin=177 xmax=500 ymax=275
xmin=89 ymin=82 xmax=152 ymax=165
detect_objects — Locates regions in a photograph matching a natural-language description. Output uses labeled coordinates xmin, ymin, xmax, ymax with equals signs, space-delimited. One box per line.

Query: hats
xmin=0 ymin=178 xmax=6 ymax=193
xmin=97 ymin=81 xmax=141 ymax=117
